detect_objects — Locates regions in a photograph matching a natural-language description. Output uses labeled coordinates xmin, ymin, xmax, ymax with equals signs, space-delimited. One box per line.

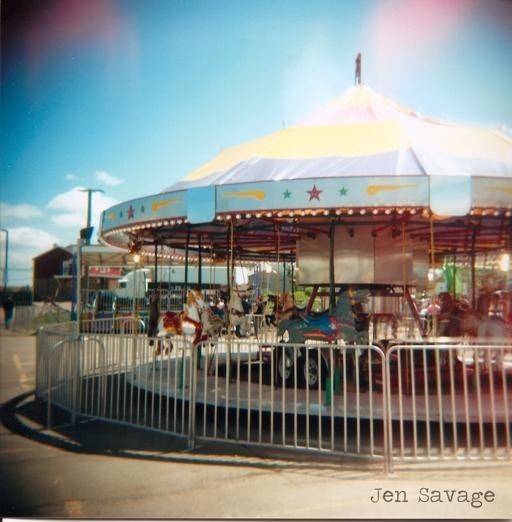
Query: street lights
xmin=0 ymin=227 xmax=7 ymax=294
xmin=78 ymin=187 xmax=104 ymax=244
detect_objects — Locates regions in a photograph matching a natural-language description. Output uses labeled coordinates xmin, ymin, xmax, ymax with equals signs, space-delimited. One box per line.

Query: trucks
xmin=114 ymin=264 xmax=249 ymax=308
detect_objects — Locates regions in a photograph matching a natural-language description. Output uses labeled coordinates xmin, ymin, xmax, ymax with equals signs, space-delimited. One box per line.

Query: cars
xmin=91 ymin=289 xmax=116 ymax=306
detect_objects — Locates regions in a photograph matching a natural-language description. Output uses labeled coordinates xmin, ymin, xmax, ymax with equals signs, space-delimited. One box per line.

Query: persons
xmin=242 ymin=295 xmax=252 ymax=315
xmin=255 ymin=295 xmax=268 ymax=314
xmin=147 ymin=291 xmax=160 ymax=345
